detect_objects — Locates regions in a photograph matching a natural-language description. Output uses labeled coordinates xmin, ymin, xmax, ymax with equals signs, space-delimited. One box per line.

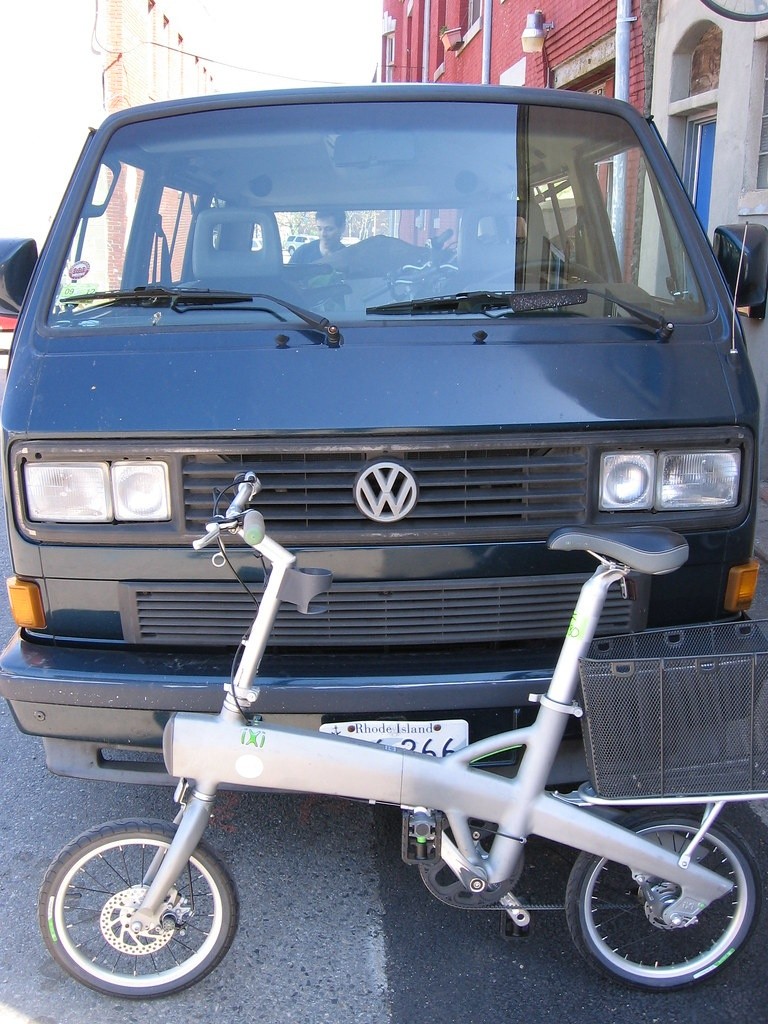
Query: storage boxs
xmin=581 ymin=617 xmax=767 ymax=796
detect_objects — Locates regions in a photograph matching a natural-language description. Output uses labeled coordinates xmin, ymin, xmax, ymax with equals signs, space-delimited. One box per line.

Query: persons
xmin=288 ymin=211 xmax=347 ymax=264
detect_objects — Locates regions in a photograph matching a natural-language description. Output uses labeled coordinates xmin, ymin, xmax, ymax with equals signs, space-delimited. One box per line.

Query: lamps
xmin=522 ymin=8 xmax=554 ymax=54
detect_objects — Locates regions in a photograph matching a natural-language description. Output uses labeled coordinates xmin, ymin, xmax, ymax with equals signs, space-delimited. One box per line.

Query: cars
xmin=340 ymin=236 xmax=358 ymax=247
xmin=251 ymin=238 xmax=262 ymax=251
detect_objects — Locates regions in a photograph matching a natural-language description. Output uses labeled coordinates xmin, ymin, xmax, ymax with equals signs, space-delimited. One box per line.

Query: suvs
xmin=285 ymin=234 xmax=318 ymax=257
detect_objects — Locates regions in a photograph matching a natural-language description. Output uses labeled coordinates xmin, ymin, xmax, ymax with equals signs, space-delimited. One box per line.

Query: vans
xmin=0 ymin=85 xmax=762 ymax=795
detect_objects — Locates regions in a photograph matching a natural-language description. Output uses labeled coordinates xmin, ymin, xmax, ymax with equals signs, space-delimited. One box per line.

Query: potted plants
xmin=440 ymin=26 xmax=463 ymax=50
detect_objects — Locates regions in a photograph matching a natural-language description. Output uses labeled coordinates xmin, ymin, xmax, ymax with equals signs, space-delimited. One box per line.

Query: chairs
xmin=192 ymin=207 xmax=284 ymax=281
xmin=458 ymin=202 xmax=555 ymax=292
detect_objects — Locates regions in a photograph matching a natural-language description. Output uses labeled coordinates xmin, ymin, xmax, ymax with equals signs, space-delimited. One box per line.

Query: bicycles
xmin=34 ymin=469 xmax=767 ymax=1003
